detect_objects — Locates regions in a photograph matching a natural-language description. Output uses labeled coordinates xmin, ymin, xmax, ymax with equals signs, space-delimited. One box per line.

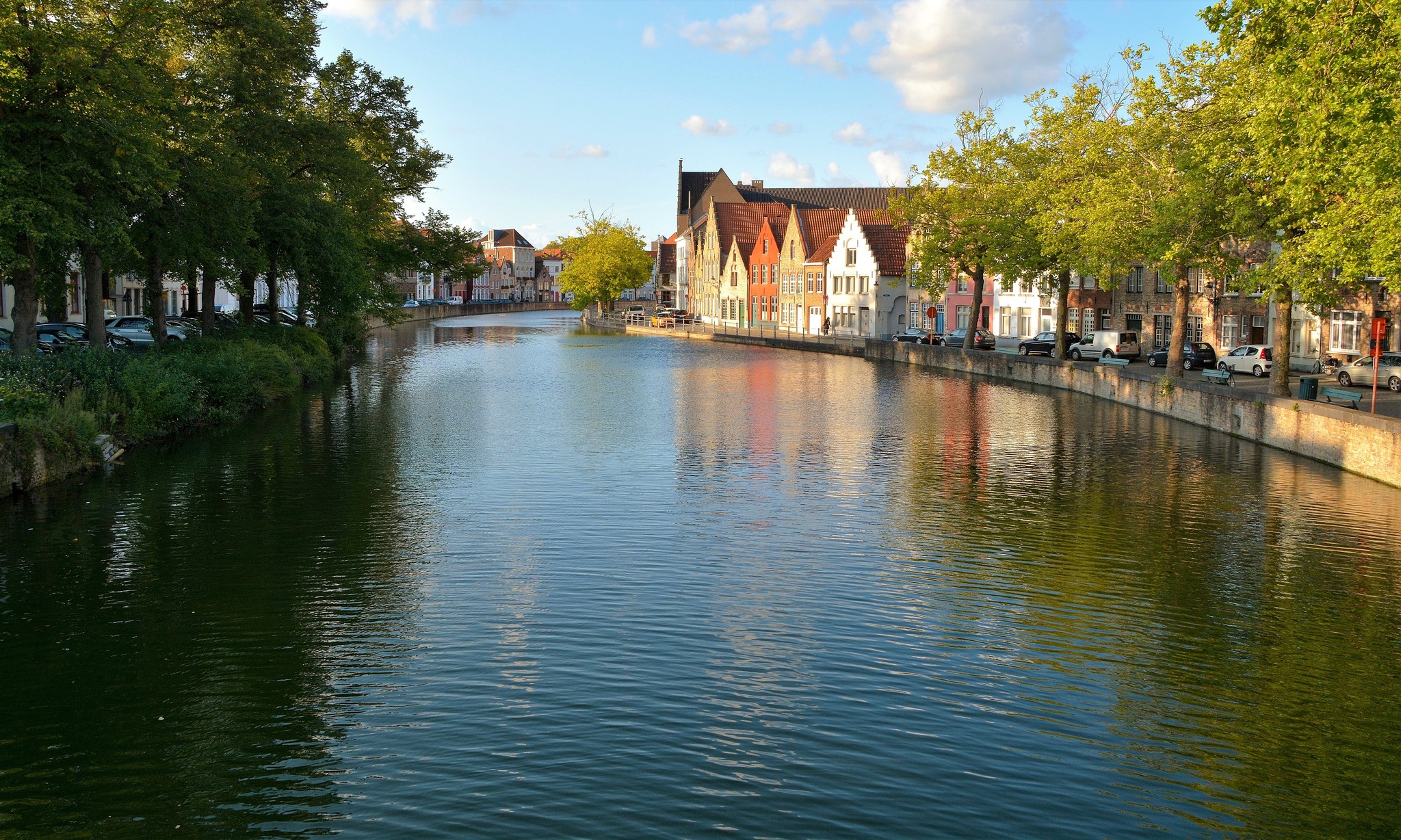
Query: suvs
xmin=650 ymin=301 xmax=689 ymax=328
xmin=563 ymin=297 xmax=574 ymax=302
xmin=939 ymin=327 xmax=996 ymax=348
xmin=1019 ymin=331 xmax=1082 ymax=359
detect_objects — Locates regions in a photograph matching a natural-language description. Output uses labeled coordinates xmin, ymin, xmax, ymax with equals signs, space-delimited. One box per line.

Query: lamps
xmin=946 ymin=297 xmax=949 ymax=301
xmin=727 ymin=295 xmax=729 ymax=301
xmin=874 ymin=281 xmax=879 ymax=297
xmin=710 ymin=276 xmax=719 ymax=289
xmin=736 ymin=295 xmax=738 ymax=300
xmin=1204 ymin=281 xmax=1219 ymax=306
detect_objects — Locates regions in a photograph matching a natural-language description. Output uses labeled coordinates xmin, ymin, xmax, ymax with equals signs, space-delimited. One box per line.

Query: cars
xmin=620 ymin=308 xmax=627 ymax=318
xmin=1336 ymin=351 xmax=1401 ymax=393
xmin=615 ymin=297 xmax=632 ymax=301
xmin=892 ymin=328 xmax=943 ymax=345
xmin=374 ymin=296 xmax=515 ymax=309
xmin=1216 ymin=344 xmax=1275 ymax=376
xmin=636 ymin=297 xmax=651 ymax=301
xmin=1145 ymin=341 xmax=1217 ymax=370
xmin=0 ymin=304 xmax=318 ymax=356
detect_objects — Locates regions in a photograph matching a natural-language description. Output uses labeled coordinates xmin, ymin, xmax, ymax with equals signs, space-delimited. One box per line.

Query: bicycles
xmin=1311 ymin=350 xmax=1336 ymax=375
xmin=1215 ymin=364 xmax=1236 ymax=388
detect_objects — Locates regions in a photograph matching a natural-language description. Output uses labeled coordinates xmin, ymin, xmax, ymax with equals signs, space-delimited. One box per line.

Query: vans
xmin=627 ymin=305 xmax=646 ymax=322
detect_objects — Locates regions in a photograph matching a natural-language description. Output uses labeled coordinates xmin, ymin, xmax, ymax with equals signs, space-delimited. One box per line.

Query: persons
xmin=823 ymin=317 xmax=831 ymax=336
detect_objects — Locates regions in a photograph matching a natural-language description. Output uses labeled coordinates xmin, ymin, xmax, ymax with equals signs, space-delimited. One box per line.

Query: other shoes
xmin=827 ymin=334 xmax=829 ymax=336
xmin=825 ymin=334 xmax=827 ymax=336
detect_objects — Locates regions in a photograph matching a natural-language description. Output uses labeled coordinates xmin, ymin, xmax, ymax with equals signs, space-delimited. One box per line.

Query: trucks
xmin=1068 ymin=330 xmax=1141 ymax=361
